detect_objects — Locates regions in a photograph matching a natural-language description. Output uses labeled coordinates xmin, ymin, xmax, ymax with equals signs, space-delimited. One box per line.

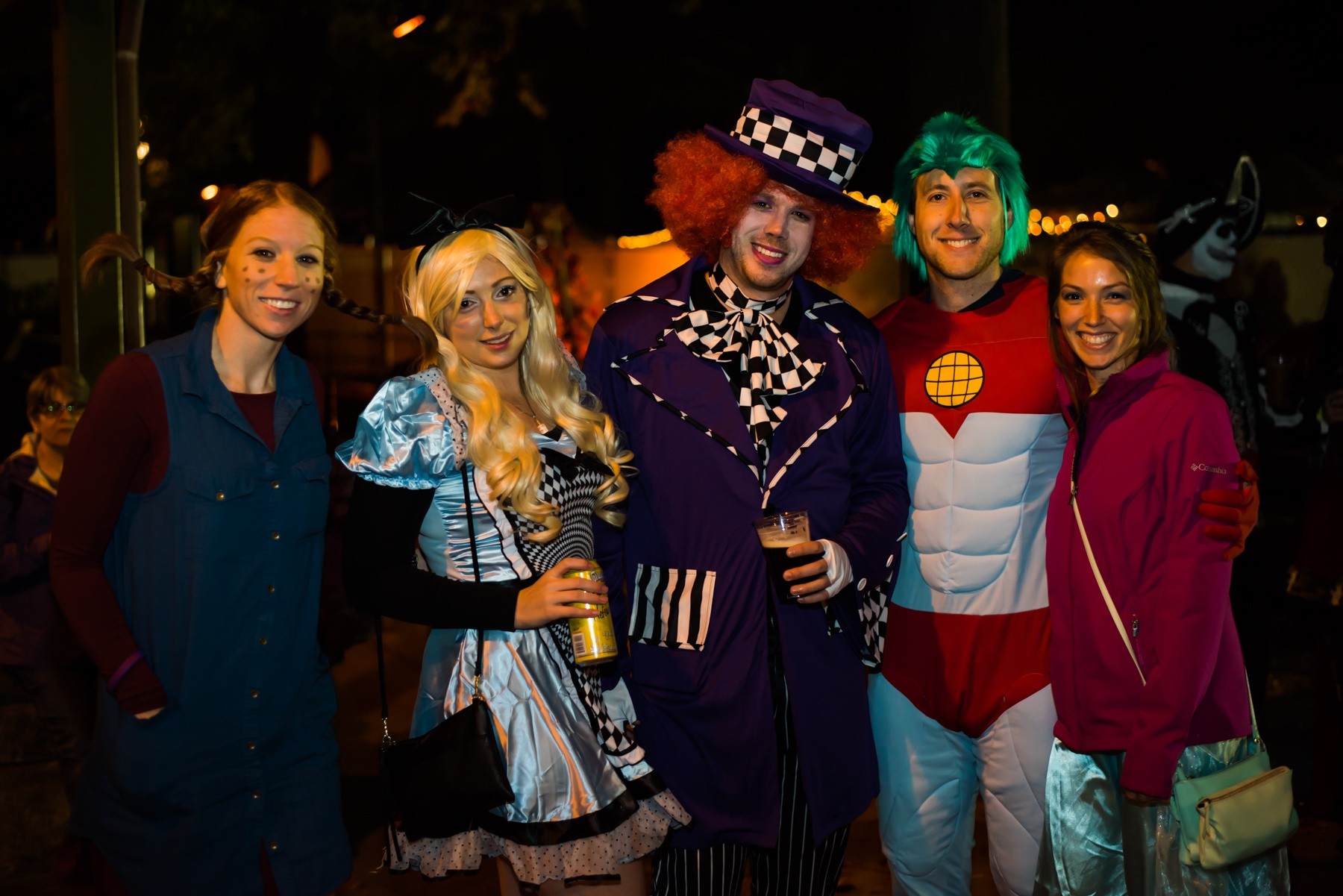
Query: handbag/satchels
xmin=1161 ymin=749 xmax=1305 ymax=873
xmin=373 ymin=694 xmax=515 ymax=831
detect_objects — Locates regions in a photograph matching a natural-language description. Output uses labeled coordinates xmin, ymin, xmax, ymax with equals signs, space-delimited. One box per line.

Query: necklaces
xmin=500 ymin=392 xmax=549 ymax=435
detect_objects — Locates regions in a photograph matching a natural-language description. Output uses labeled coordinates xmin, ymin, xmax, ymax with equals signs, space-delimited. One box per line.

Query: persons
xmin=1142 ymin=155 xmax=1343 ymax=825
xmin=330 ymin=222 xmax=692 ymax=896
xmin=582 ymin=73 xmax=913 ymax=896
xmin=0 ymin=362 xmax=104 ymax=883
xmin=46 ymin=178 xmax=357 ymax=896
xmin=1043 ymin=221 xmax=1291 ymax=894
xmin=860 ymin=111 xmax=1262 ymax=895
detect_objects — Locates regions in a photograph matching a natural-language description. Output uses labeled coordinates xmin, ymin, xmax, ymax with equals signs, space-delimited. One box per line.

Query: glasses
xmin=37 ymin=399 xmax=85 ymax=419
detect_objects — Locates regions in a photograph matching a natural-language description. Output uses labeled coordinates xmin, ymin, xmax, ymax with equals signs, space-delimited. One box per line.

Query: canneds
xmin=558 ymin=563 xmax=618 ymax=665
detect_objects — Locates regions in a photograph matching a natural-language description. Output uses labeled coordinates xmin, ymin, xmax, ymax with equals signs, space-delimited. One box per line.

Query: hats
xmin=701 ymin=77 xmax=884 ymax=219
xmin=1152 ymin=177 xmax=1242 ymax=269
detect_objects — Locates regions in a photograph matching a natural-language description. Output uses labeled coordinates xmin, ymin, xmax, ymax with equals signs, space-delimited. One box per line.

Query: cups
xmin=754 ymin=510 xmax=816 ymax=601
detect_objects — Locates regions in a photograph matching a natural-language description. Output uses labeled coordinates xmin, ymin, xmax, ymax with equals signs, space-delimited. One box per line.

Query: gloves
xmin=1197 ymin=459 xmax=1261 ymax=562
